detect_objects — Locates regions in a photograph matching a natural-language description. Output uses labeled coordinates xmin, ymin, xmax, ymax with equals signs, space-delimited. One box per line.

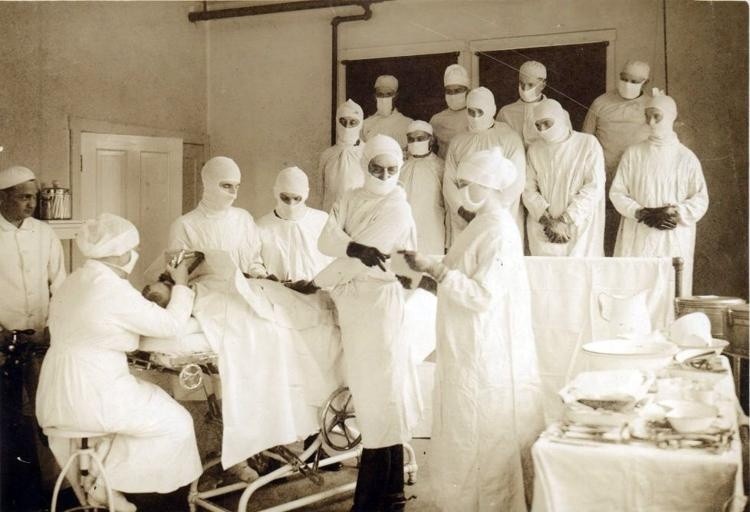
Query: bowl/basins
xmin=582 ymin=341 xmax=672 ymax=370
xmin=680 ymin=338 xmax=730 ymax=355
xmin=666 ymin=408 xmax=715 ymax=431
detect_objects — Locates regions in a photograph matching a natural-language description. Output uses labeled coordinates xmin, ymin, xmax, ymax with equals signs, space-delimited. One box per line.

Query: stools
xmin=42 ymin=425 xmax=118 ymax=512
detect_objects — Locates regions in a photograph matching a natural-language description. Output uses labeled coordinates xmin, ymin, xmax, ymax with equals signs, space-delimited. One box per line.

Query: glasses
xmin=371 ymin=162 xmax=398 ymax=175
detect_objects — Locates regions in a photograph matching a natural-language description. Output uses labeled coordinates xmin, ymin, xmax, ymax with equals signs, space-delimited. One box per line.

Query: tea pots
xmin=596 ymin=288 xmax=652 ymax=339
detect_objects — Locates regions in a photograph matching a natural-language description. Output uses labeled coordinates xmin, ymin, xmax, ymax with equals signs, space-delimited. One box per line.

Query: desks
xmin=531 ymin=354 xmax=748 ymax=512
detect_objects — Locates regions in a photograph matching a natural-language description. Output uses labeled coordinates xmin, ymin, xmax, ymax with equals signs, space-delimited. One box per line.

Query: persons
xmin=140 ymin=276 xmax=192 ymax=331
xmin=521 ymin=97 xmax=607 ymax=258
xmin=0 ymin=165 xmax=66 ymax=512
xmin=165 ymin=156 xmax=279 ymax=482
xmin=396 ymin=118 xmax=445 ymax=258
xmin=608 ymin=95 xmax=710 ymax=297
xmin=428 ymin=64 xmax=472 ymax=160
xmin=317 ymin=134 xmax=420 ymax=512
xmin=583 ymin=59 xmax=653 ymax=256
xmin=315 ymin=98 xmax=366 ymax=214
xmin=495 ymin=60 xmax=573 ymax=150
xmin=442 ymin=85 xmax=528 ymax=257
xmin=34 ymin=212 xmax=205 ymax=512
xmin=388 ymin=145 xmax=536 ymax=512
xmin=359 ymin=74 xmax=417 ymax=152
xmin=256 ymin=166 xmax=338 ymax=284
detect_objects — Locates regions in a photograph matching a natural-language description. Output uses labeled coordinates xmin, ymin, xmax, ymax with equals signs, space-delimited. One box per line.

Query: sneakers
xmin=87 ymin=484 xmax=136 ymax=511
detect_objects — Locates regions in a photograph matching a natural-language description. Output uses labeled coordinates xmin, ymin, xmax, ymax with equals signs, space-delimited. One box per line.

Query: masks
xmin=377 ymin=97 xmax=393 ymax=118
xmin=101 ymin=250 xmax=139 ymax=275
xmin=618 ymin=78 xmax=648 ymax=100
xmin=456 ymin=182 xmax=488 ymax=213
xmin=519 ymin=78 xmax=543 ymax=103
xmin=408 ymin=139 xmax=431 ymax=156
xmin=445 ymin=93 xmax=467 ymax=111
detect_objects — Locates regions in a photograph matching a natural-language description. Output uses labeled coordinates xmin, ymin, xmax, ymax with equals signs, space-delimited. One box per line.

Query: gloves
xmin=347 ymin=241 xmax=391 ymax=272
xmin=637 ymin=206 xmax=677 ymax=230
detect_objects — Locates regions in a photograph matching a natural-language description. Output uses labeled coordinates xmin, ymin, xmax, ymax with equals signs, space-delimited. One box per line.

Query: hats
xmin=623 ymin=59 xmax=649 ymax=79
xmin=406 ymin=120 xmax=434 ymax=134
xmin=520 ymin=61 xmax=547 ymax=80
xmin=443 ymin=64 xmax=469 ymax=90
xmin=374 ymin=75 xmax=398 ymax=93
xmin=1 ymin=166 xmax=36 ymax=188
xmin=457 ymin=150 xmax=516 ymax=190
xmin=75 ymin=214 xmax=139 ymax=259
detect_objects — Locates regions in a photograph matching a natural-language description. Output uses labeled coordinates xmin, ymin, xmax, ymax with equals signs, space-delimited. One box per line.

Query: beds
xmin=127 ymin=258 xmax=457 ymax=512
